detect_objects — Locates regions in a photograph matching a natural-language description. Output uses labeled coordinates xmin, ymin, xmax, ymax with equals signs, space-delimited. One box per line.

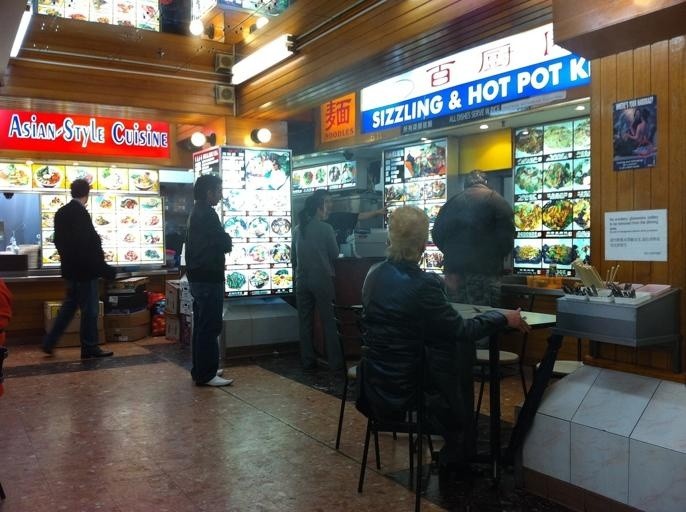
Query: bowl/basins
xmin=34 ymin=168 xmax=64 ymax=187
xmin=132 ymin=171 xmax=158 ymax=190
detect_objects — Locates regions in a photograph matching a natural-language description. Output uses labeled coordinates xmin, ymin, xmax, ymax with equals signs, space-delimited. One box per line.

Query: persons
xmin=290 ymin=196 xmax=349 ymax=376
xmin=430 ymin=170 xmax=515 ymax=383
xmin=0 ymin=276 xmax=17 ymax=501
xmin=37 ymin=179 xmax=115 ymax=359
xmin=360 ymin=207 xmax=533 ymax=482
xmin=314 ymin=189 xmax=391 ymax=252
xmin=185 ymin=174 xmax=235 ymax=387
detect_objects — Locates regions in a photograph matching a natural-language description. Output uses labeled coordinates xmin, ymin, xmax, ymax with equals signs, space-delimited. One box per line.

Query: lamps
xmin=251 ymin=127 xmax=271 ymax=146
xmin=191 ymin=129 xmax=216 ymax=148
xmin=230 ymin=35 xmax=294 ymax=85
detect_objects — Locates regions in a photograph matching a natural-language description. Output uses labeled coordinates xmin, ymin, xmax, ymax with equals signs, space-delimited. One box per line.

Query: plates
xmin=542 ymin=198 xmax=573 ymax=230
xmin=68 ymin=168 xmax=95 ymax=187
xmin=245 ymin=152 xmax=288 ymax=190
xmin=224 ymin=216 xmax=291 ymax=287
xmin=99 ymin=171 xmax=126 ymax=189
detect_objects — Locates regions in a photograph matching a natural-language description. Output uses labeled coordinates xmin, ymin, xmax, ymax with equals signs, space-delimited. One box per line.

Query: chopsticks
xmin=574 ymin=263 xmax=620 ymax=288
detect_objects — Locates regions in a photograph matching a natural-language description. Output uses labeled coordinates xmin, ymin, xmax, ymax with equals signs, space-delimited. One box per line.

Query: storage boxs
xmin=43 ymin=276 xmax=150 ymax=349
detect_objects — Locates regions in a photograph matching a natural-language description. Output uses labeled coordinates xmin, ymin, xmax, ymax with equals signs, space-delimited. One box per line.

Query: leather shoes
xmin=192 ymin=369 xmax=233 ymax=386
xmin=81 ymin=348 xmax=114 ymax=358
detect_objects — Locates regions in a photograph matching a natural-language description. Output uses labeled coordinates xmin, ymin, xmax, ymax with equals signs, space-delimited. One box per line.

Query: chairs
xmin=329 ymin=298 xmax=447 ymax=511
xmin=475 ymin=286 xmax=588 ymax=447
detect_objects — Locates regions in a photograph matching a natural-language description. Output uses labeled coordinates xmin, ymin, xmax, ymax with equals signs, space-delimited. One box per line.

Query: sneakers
xmin=42 ymin=342 xmax=52 ymax=355
xmin=304 ymin=363 xmax=345 ymax=383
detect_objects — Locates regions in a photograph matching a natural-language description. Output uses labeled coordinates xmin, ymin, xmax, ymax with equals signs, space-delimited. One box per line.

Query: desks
xmin=443 ymin=301 xmax=557 ymax=489
